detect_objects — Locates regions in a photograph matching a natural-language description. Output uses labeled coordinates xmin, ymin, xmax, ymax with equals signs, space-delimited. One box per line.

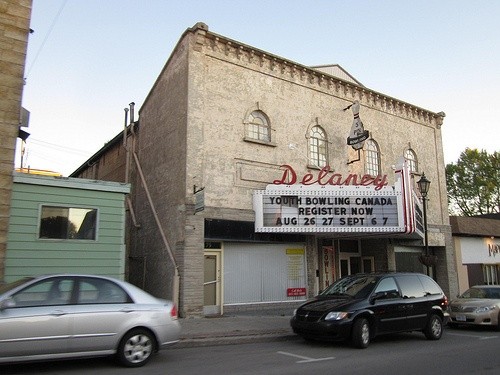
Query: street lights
xmin=415 ymin=171 xmax=433 ymax=279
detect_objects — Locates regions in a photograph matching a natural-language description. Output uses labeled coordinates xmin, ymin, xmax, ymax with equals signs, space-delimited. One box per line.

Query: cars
xmin=0 ymin=273 xmax=181 ymax=367
xmin=448 ymin=285 xmax=500 ymax=330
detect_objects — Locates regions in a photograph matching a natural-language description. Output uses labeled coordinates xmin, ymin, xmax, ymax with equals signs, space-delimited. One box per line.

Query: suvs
xmin=290 ymin=272 xmax=448 ymax=348
